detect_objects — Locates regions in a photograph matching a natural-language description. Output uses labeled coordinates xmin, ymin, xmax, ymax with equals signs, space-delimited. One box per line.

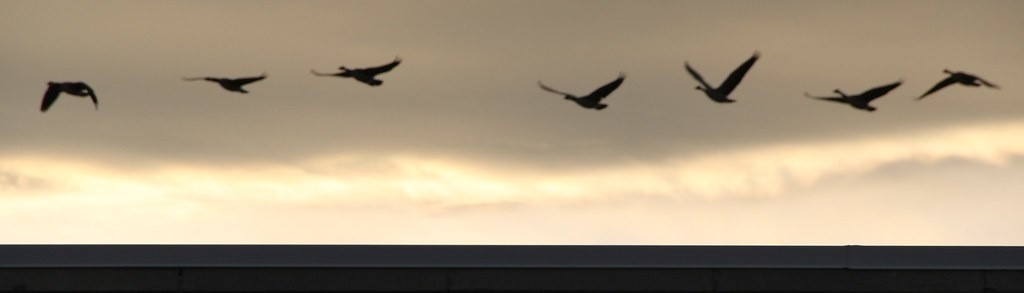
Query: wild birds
xmin=805 ymin=78 xmax=906 ymax=114
xmin=685 ymin=49 xmax=763 ymax=104
xmin=918 ymin=67 xmax=1002 ymax=104
xmin=184 ymin=74 xmax=268 ymax=95
xmin=311 ymin=55 xmax=403 ymax=88
xmin=532 ymin=67 xmax=627 ymax=112
xmin=40 ymin=80 xmax=98 ymax=116
